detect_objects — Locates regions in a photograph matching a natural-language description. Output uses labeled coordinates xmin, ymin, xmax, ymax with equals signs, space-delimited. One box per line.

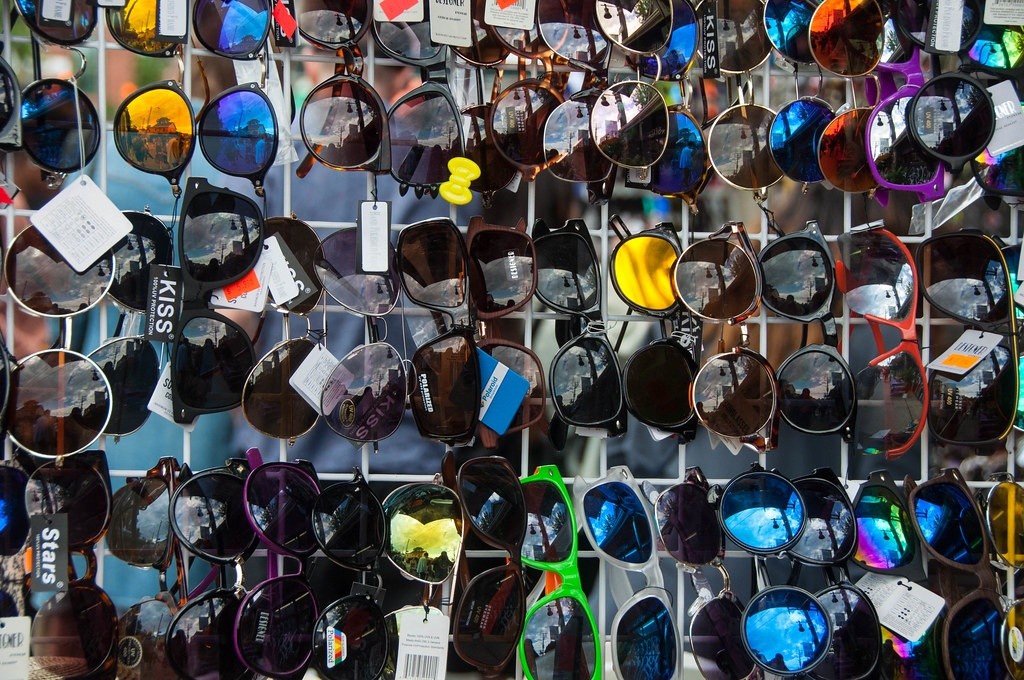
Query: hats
xmin=261 ymin=133 xmax=267 ymax=137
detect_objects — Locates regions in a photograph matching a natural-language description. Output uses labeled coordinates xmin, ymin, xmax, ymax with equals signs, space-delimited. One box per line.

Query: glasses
xmin=0 ymin=1 xmax=1016 ymax=679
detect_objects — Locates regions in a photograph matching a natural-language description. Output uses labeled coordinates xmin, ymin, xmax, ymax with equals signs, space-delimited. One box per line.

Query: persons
xmin=28 ymin=291 xmax=55 ymax=315
xmin=226 ymin=132 xmax=271 ymax=170
xmin=236 ymin=0 xmax=481 ymax=475
xmin=34 ymin=410 xmax=53 ymax=452
xmin=30 ymin=34 xmax=236 ymax=612
xmin=136 ymin=37 xmax=147 ymax=52
xmin=393 ymin=551 xmax=454 ymax=580
xmin=132 ymin=131 xmax=181 ymax=168
xmin=587 ymin=184 xmax=902 ymax=634
xmin=678 ymin=141 xmax=695 ymax=188
